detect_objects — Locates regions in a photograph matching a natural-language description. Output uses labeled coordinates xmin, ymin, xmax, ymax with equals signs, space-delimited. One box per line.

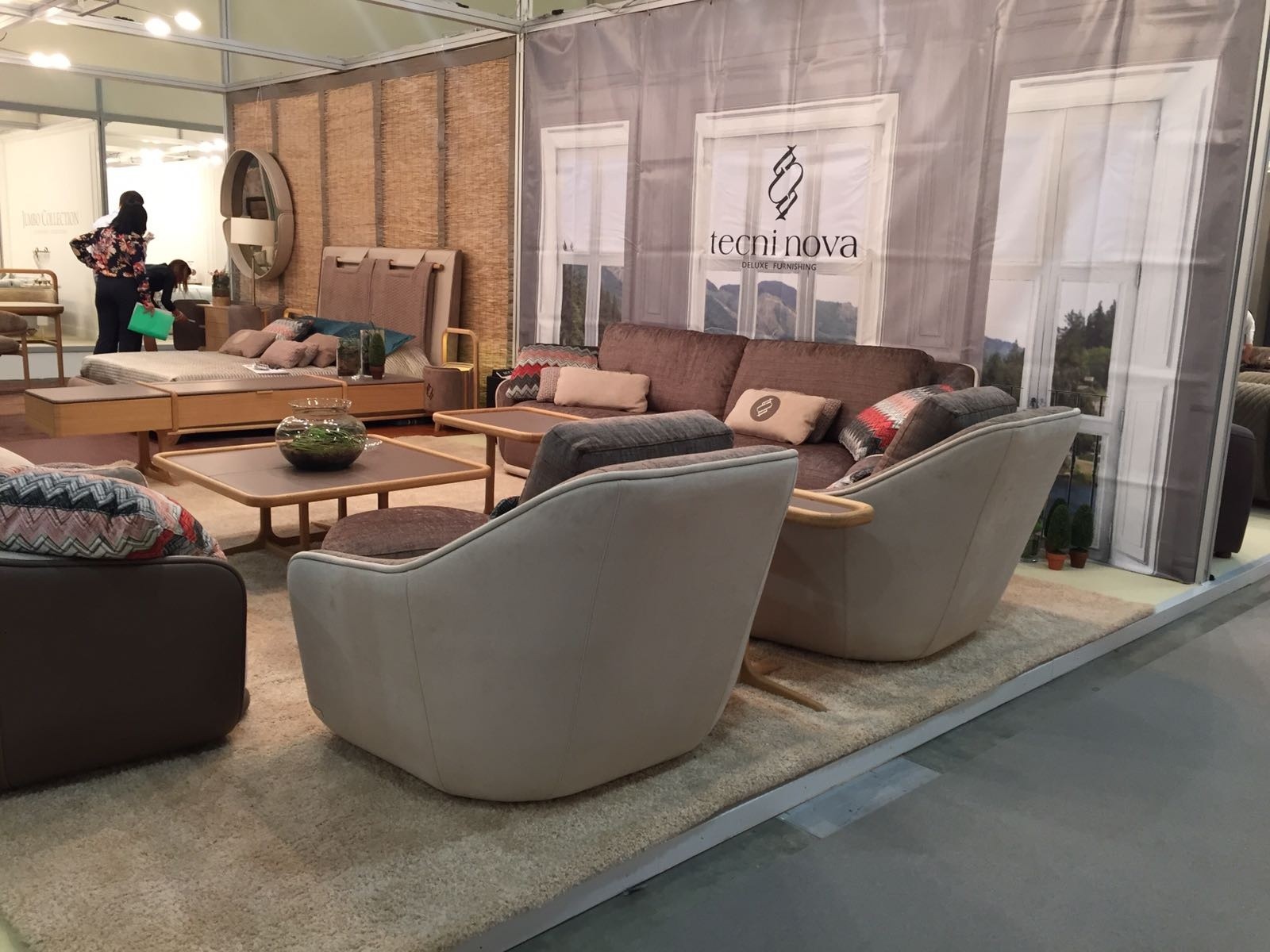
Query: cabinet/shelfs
xmin=23 ymin=372 xmax=426 ymax=487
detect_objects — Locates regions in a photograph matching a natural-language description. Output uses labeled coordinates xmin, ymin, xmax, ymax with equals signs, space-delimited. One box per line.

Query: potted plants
xmin=1020 ymin=503 xmax=1094 ymax=572
xmin=208 ymin=268 xmax=230 ymax=288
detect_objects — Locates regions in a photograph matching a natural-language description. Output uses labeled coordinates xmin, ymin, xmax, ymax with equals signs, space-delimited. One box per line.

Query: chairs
xmin=0 ymin=310 xmax=28 ymax=390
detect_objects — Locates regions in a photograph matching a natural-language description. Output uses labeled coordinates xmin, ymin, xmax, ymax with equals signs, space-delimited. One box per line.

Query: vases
xmin=336 ymin=329 xmax=386 ymax=380
xmin=276 ymin=397 xmax=367 ymax=471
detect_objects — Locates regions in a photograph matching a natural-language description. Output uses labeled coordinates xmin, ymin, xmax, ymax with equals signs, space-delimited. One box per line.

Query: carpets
xmin=0 ymin=432 xmax=1156 ymax=952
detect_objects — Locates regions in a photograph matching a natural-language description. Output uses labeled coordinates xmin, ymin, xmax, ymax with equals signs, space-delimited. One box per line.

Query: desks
xmin=0 ymin=301 xmax=65 ymax=390
xmin=151 ymin=406 xmax=592 ymax=560
xmin=739 ymin=488 xmax=876 ymax=713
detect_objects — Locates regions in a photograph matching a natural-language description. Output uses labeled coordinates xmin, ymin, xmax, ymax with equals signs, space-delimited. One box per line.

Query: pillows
xmin=724 ymin=384 xmax=959 ymax=462
xmin=218 ymin=317 xmax=417 ymax=369
xmin=0 ymin=463 xmax=230 ymax=562
xmin=504 ymin=342 xmax=651 ymax=414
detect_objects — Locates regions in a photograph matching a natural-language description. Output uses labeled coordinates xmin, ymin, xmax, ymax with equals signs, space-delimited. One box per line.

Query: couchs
xmin=286 ymin=404 xmax=799 ymax=803
xmin=751 ymin=387 xmax=1082 ymax=664
xmin=0 ymin=447 xmax=247 ymax=797
xmin=495 ymin=322 xmax=1018 ymax=479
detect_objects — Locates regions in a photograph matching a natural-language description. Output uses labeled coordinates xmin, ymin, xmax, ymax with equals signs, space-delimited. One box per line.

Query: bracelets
xmin=176 ymin=311 xmax=183 ymax=320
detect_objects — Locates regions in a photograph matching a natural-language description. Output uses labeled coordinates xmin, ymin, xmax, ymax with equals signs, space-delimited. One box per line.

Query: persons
xmin=92 ymin=191 xmax=144 ymax=231
xmin=69 ymin=200 xmax=155 ymax=355
xmin=144 ymin=260 xmax=192 ymax=351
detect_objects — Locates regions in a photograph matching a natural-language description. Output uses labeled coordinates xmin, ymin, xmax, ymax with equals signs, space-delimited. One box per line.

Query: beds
xmin=69 ymin=246 xmax=462 ymax=386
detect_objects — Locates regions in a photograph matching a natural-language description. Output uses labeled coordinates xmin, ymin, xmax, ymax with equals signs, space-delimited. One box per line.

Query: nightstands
xmin=195 ymin=304 xmax=281 ymax=350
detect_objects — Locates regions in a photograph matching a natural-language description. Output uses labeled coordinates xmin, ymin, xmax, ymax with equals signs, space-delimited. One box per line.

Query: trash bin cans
xmin=486 ymin=369 xmax=515 ymax=444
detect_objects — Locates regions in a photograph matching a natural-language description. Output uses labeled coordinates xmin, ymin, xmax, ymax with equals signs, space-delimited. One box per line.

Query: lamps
xmin=231 ymin=217 xmax=276 ymax=306
